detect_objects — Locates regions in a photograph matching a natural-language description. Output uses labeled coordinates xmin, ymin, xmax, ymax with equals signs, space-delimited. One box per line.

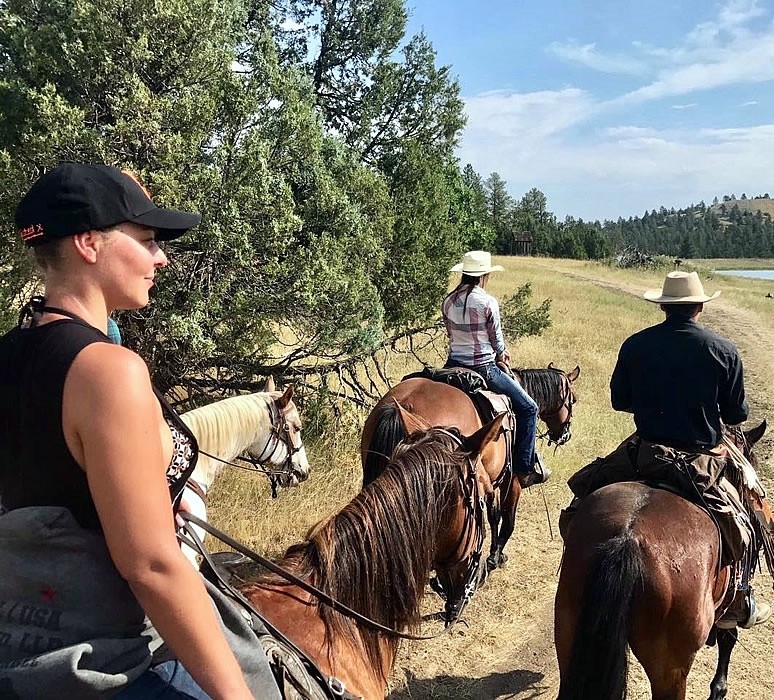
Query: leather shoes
xmin=715 ymin=603 xmax=771 ymax=628
xmin=518 ymin=468 xmax=552 ymax=489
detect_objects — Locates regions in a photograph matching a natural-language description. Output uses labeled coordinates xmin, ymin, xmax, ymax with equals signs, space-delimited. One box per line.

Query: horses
xmin=359 ymin=360 xmax=581 ymax=569
xmin=175 ymin=373 xmax=311 ymax=567
xmin=553 ymin=419 xmax=770 ymax=700
xmin=218 ymin=395 xmax=511 ymax=700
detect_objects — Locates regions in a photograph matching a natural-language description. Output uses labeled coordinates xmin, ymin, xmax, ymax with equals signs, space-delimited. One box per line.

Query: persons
xmin=0 ymin=165 xmax=256 ymax=699
xmin=566 ymin=272 xmax=771 ymax=628
xmin=442 ymin=251 xmax=552 ymax=487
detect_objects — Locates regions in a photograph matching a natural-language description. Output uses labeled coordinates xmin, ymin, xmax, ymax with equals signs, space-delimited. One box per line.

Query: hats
xmin=643 ymin=272 xmax=721 ymax=304
xmin=449 ymin=251 xmax=504 ymax=277
xmin=15 ymin=163 xmax=200 ymax=247
